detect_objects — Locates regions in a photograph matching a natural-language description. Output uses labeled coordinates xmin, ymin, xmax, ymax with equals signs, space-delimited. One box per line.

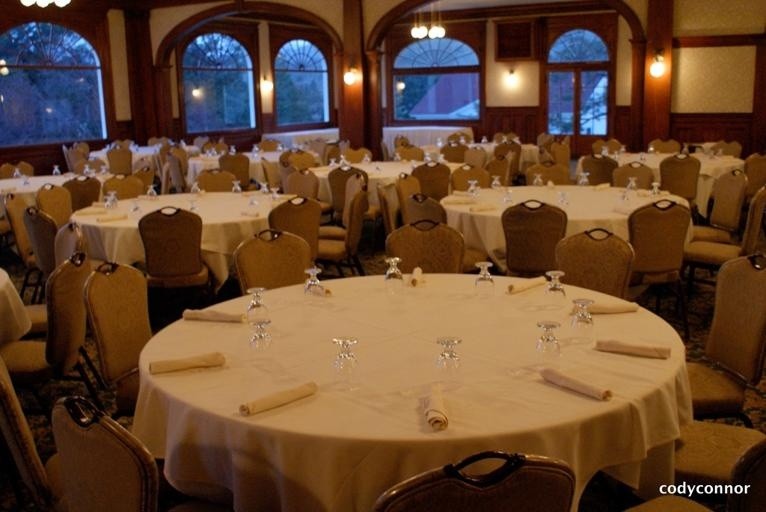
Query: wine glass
xmin=245 ymin=286 xmax=272 ymax=350
xmin=382 ymin=256 xmax=405 ymax=296
xmin=567 ymin=295 xmax=597 ymax=345
xmin=543 ymin=270 xmax=568 ymax=311
xmin=471 ymin=260 xmax=495 ymax=298
xmin=332 ymin=336 xmax=364 ymax=391
xmin=533 ymin=318 xmax=561 ymax=371
xmin=301 ymin=269 xmax=327 ymax=307
xmin=10 ymin=136 xmax=430 ymax=224
xmin=431 ymin=131 xmax=728 ymax=224
xmin=432 ymin=336 xmax=465 ymax=393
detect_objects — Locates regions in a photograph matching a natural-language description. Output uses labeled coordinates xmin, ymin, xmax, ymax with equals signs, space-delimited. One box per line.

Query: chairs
xmin=0 ymin=129 xmax=766 ymax=511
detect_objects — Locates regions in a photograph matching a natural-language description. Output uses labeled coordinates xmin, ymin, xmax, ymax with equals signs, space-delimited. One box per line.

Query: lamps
xmin=260 ymin=75 xmax=275 ymax=93
xmin=344 ymin=60 xmax=360 ymax=86
xmin=409 ymin=1 xmax=446 ymax=41
xmin=648 ymin=47 xmax=669 ymax=79
